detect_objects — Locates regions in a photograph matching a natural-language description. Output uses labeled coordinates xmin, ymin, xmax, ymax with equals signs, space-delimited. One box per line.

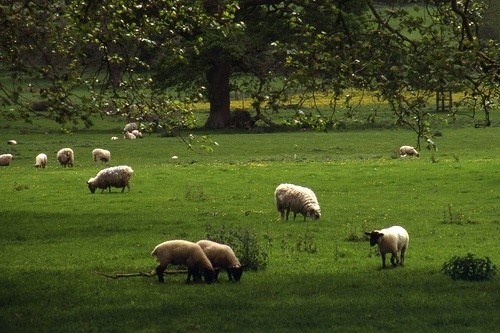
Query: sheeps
xmin=151 ymin=239 xmax=245 ymax=285
xmin=0 ymin=153 xmax=15 ymax=166
xmin=400 ymin=146 xmax=420 ymax=158
xmin=57 ymin=147 xmax=75 ymax=168
xmin=275 ymin=183 xmax=322 ymax=223
xmin=364 ymin=225 xmax=410 ymax=269
xmin=87 ymin=164 xmax=135 ymax=194
xmin=92 ymin=148 xmax=111 ymax=163
xmin=110 ymin=122 xmax=142 ymax=140
xmin=33 ymin=153 xmax=48 ymax=169
